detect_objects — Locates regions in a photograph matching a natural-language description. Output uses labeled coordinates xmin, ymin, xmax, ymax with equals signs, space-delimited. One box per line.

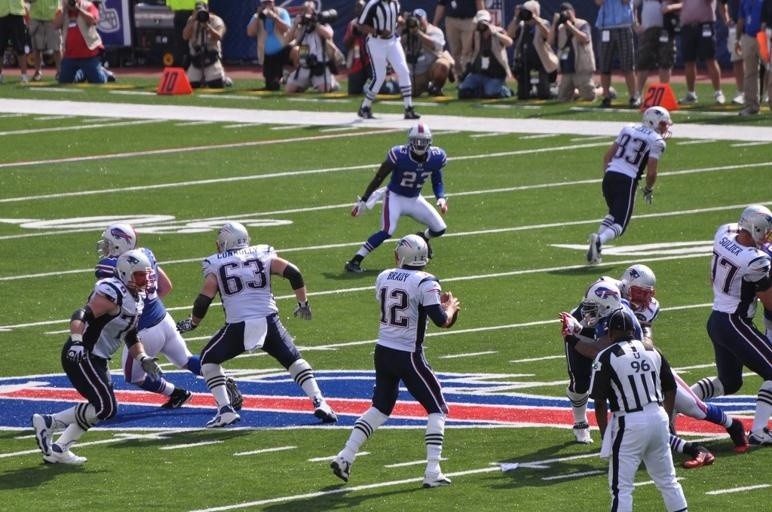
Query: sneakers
xmin=312 ymin=399 xmax=339 ymax=422
xmin=600 ymin=86 xmax=641 ymax=109
xmin=32 ymin=414 xmax=56 ymax=456
xmin=345 ymin=261 xmax=367 ymax=273
xmin=21 ymin=71 xmax=60 ymax=82
xmin=224 ymin=77 xmax=233 ymax=86
xmin=161 ymin=379 xmax=244 ymax=428
xmin=588 ymin=233 xmax=602 ymax=265
xmin=683 ymin=447 xmax=716 ymax=468
xmin=405 ymin=107 xmax=420 ymax=119
xmin=330 ymin=457 xmax=351 ymax=482
xmin=43 ymin=444 xmax=88 ymax=464
xmin=416 ymin=232 xmax=432 ymax=259
xmin=573 ymin=423 xmax=594 ymax=444
xmin=423 ymin=471 xmax=452 ymax=488
xmin=678 ymin=94 xmax=762 ymax=117
xmin=748 ymin=428 xmax=772 ymax=447
xmin=359 ymin=104 xmax=374 ymax=119
xmin=730 ymin=419 xmax=749 ymax=454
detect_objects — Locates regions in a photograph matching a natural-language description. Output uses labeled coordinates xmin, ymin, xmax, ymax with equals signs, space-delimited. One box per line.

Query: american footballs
xmin=440 ymin=292 xmax=458 ymax=328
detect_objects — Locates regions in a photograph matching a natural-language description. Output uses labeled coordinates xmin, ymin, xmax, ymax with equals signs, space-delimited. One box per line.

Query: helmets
xmin=642 ymin=106 xmax=673 ymax=141
xmin=409 ymin=123 xmax=433 ymax=155
xmin=117 ymin=250 xmax=152 ymax=295
xmin=739 ymin=206 xmax=772 ymax=249
xmin=394 ymin=234 xmax=428 ymax=270
xmin=216 ymin=222 xmax=249 ymax=253
xmin=97 ymin=223 xmax=137 ymax=259
xmin=581 ymin=264 xmax=656 ymax=331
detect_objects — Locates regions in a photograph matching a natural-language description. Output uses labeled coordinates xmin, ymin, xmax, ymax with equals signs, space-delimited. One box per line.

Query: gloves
xmin=176 ymin=318 xmax=196 ymax=334
xmin=641 ymin=186 xmax=654 ymax=204
xmin=351 ymin=200 xmax=366 ymax=218
xmin=136 ymin=353 xmax=162 ymax=379
xmin=435 ymin=198 xmax=447 ymax=216
xmin=558 ymin=312 xmax=583 ymax=337
xmin=66 ymin=334 xmax=87 ymax=364
xmin=294 ymin=298 xmax=312 ymax=320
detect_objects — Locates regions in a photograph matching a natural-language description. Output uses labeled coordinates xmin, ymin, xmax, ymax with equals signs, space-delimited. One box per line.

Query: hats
xmin=476 ymin=9 xmax=492 ymax=23
xmin=413 ymin=9 xmax=426 ymax=17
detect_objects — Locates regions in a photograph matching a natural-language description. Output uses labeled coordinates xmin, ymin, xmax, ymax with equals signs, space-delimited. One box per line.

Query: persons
xmin=244 ymin=1 xmax=342 ymax=93
xmin=344 ymin=122 xmax=448 ymax=273
xmin=583 ymin=105 xmax=671 ymax=265
xmin=175 ymin=220 xmax=337 ymax=429
xmin=555 ymin=204 xmax=771 ymax=511
xmin=51 ymin=0 xmax=116 ymax=84
xmin=31 ymin=248 xmax=163 ymax=465
xmin=1 ymin=1 xmax=30 ymax=83
xmin=330 ymin=234 xmax=461 ymax=487
xmin=94 ymin=222 xmax=244 ymax=411
xmin=341 ymin=0 xmax=771 ymax=120
xmin=182 ymin=1 xmax=235 ymax=89
xmin=26 ymin=1 xmax=65 ymax=82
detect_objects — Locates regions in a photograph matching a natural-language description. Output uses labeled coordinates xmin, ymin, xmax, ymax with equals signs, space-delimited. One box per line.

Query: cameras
xmin=519 ymin=4 xmax=532 ymax=21
xmin=196 ymin=5 xmax=209 ymax=22
xmin=259 ymin=8 xmax=268 ymax=19
xmin=406 ymin=16 xmax=420 ymax=28
xmin=560 ymin=10 xmax=570 ymax=23
xmin=477 ymin=22 xmax=488 ymax=31
xmin=298 ymin=13 xmax=312 ymax=23
xmin=68 ymin=0 xmax=76 ymax=6
